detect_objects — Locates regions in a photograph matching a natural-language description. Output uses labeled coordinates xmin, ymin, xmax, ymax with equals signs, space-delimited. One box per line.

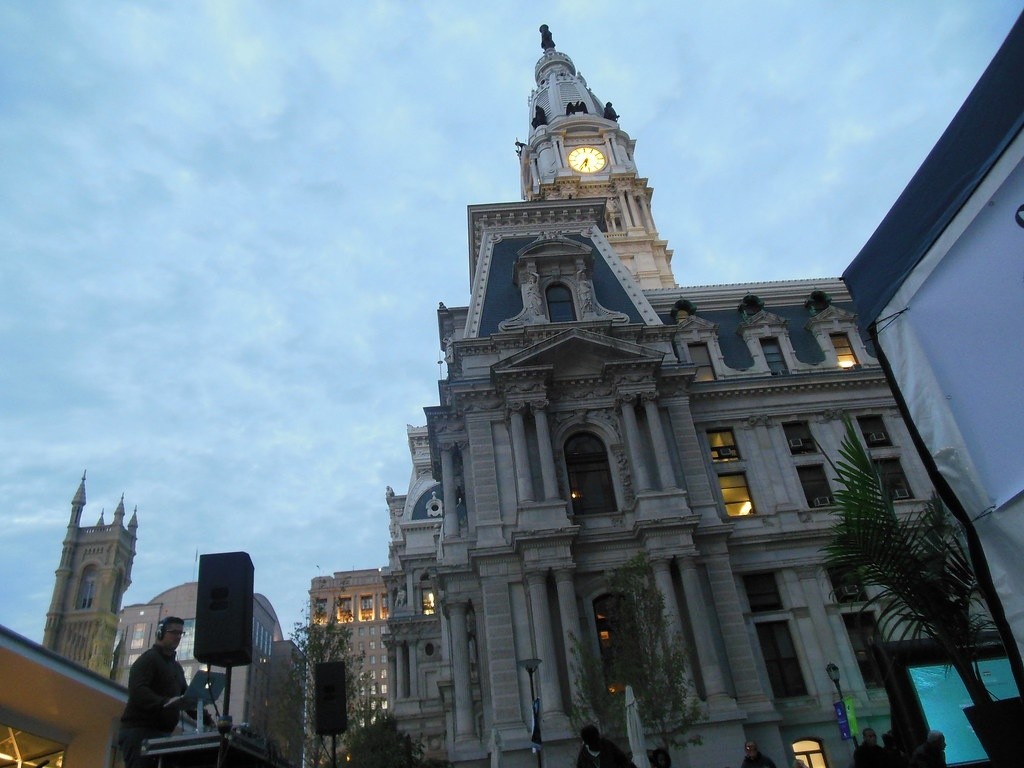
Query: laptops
xmin=163 ymin=670 xmax=225 ymax=711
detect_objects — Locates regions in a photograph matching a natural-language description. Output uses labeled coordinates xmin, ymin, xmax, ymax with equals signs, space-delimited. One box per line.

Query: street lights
xmin=515 ymin=658 xmax=543 ymax=768
xmin=824 ymin=661 xmax=859 ymax=748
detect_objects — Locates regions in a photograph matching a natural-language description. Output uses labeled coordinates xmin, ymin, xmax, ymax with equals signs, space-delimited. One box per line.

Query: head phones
xmin=156 ymin=616 xmax=171 ymax=640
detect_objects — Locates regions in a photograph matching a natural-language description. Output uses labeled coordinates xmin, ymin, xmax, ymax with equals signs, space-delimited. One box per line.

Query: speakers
xmin=194 ymin=552 xmax=255 ymax=666
xmin=315 ymin=661 xmax=346 ymax=735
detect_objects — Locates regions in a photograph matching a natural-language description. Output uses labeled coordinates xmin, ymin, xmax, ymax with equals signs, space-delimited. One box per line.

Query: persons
xmin=526 ymin=271 xmax=543 ymax=316
xmin=576 ymin=268 xmax=594 ymax=313
xmin=741 ymin=740 xmax=776 ymax=768
xmin=119 ymin=617 xmax=217 ymax=768
xmin=576 ymin=725 xmax=637 ymax=768
xmin=853 ymin=728 xmax=948 ymax=768
xmin=627 ymin=749 xmax=671 ymax=768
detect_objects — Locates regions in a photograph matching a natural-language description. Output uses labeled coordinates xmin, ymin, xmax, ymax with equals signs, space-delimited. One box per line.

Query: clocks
xmin=568 ymin=147 xmax=606 ymax=173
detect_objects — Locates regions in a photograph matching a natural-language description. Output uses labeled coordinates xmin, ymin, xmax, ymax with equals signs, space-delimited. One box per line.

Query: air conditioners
xmin=839 ymin=584 xmax=859 ymax=600
xmin=869 ymin=432 xmax=885 ymax=442
xmin=894 ymin=488 xmax=909 ymax=499
xmin=850 ymin=363 xmax=863 ymax=370
xmin=813 ymin=496 xmax=830 ymax=507
xmin=779 ymin=369 xmax=790 ymax=375
xmin=788 ymin=438 xmax=803 ymax=449
xmin=718 ymin=448 xmax=733 ymax=457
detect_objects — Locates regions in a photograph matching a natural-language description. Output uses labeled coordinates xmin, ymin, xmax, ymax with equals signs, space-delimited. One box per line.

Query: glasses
xmin=746 ymin=746 xmax=756 ymax=750
xmin=163 ymin=630 xmax=185 ymax=637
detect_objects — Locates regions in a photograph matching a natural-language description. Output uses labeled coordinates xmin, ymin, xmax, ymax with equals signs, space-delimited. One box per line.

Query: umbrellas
xmin=624 ymin=685 xmax=650 ymax=768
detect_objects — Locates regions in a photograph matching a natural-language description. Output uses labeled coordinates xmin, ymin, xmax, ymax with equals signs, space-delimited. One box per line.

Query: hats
xmin=927 ymin=729 xmax=941 ymax=743
